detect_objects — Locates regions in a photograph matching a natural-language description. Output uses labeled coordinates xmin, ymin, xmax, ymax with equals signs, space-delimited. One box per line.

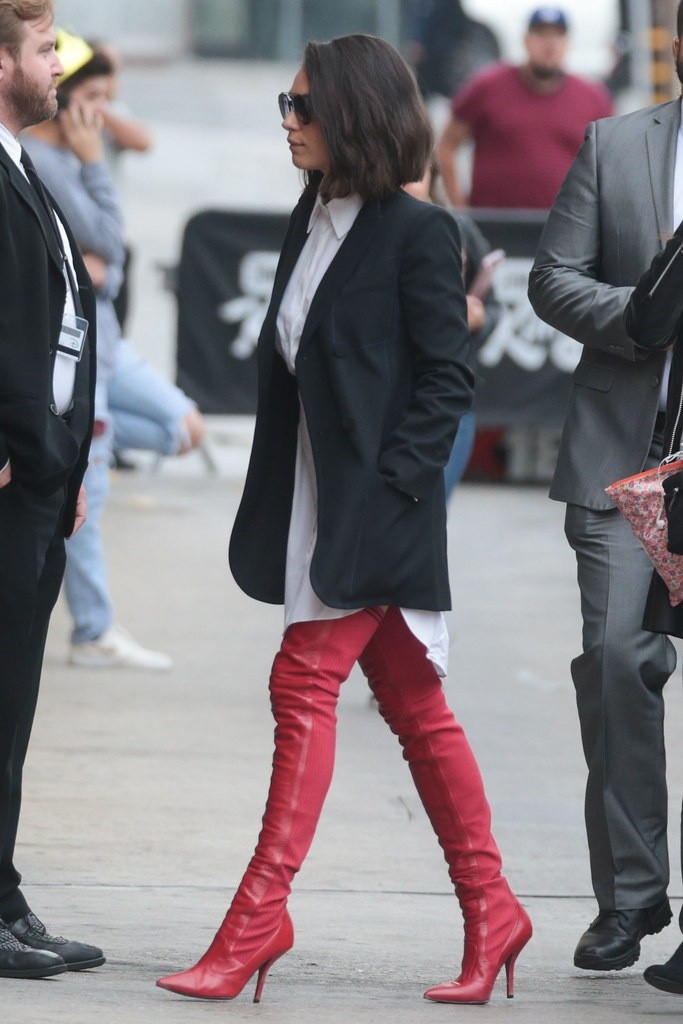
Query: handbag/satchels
xmin=604 ymin=461 xmax=683 ymax=607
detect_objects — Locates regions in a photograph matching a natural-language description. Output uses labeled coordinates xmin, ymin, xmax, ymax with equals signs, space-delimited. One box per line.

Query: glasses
xmin=277 ymin=93 xmax=313 ymax=124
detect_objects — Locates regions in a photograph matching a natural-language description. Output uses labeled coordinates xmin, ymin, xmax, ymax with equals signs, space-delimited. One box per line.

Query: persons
xmin=430 ymin=6 xmax=618 ymax=484
xmin=155 ymin=33 xmax=534 ymax=1005
xmin=1 ymin=0 xmax=205 ymax=980
xmin=527 ymin=0 xmax=683 ymax=995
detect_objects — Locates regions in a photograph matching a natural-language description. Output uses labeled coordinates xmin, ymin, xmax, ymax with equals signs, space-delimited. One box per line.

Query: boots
xmin=156 ymin=601 xmax=387 ymax=1003
xmin=357 ymin=608 xmax=533 ymax=1002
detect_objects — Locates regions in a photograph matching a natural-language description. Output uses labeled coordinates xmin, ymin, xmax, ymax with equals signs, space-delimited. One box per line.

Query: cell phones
xmin=52 ymin=92 xmax=72 ymax=109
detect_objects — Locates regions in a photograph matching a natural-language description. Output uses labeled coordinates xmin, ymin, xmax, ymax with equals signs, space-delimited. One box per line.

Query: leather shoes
xmin=7 ymin=912 xmax=105 ymax=969
xmin=644 ymin=941 xmax=683 ymax=992
xmin=572 ymin=896 xmax=673 ymax=972
xmin=0 ymin=919 xmax=67 ymax=978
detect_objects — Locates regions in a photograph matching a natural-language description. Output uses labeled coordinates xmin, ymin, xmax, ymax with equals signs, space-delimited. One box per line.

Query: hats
xmin=528 ymin=8 xmax=566 ymax=32
xmin=55 ymin=28 xmax=93 ymax=88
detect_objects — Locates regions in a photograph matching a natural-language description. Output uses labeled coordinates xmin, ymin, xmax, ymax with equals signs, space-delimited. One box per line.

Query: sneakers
xmin=64 ymin=627 xmax=168 ymax=672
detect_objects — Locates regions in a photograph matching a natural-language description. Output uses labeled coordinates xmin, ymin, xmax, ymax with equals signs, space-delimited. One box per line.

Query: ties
xmin=20 ymin=144 xmax=89 ymax=447
